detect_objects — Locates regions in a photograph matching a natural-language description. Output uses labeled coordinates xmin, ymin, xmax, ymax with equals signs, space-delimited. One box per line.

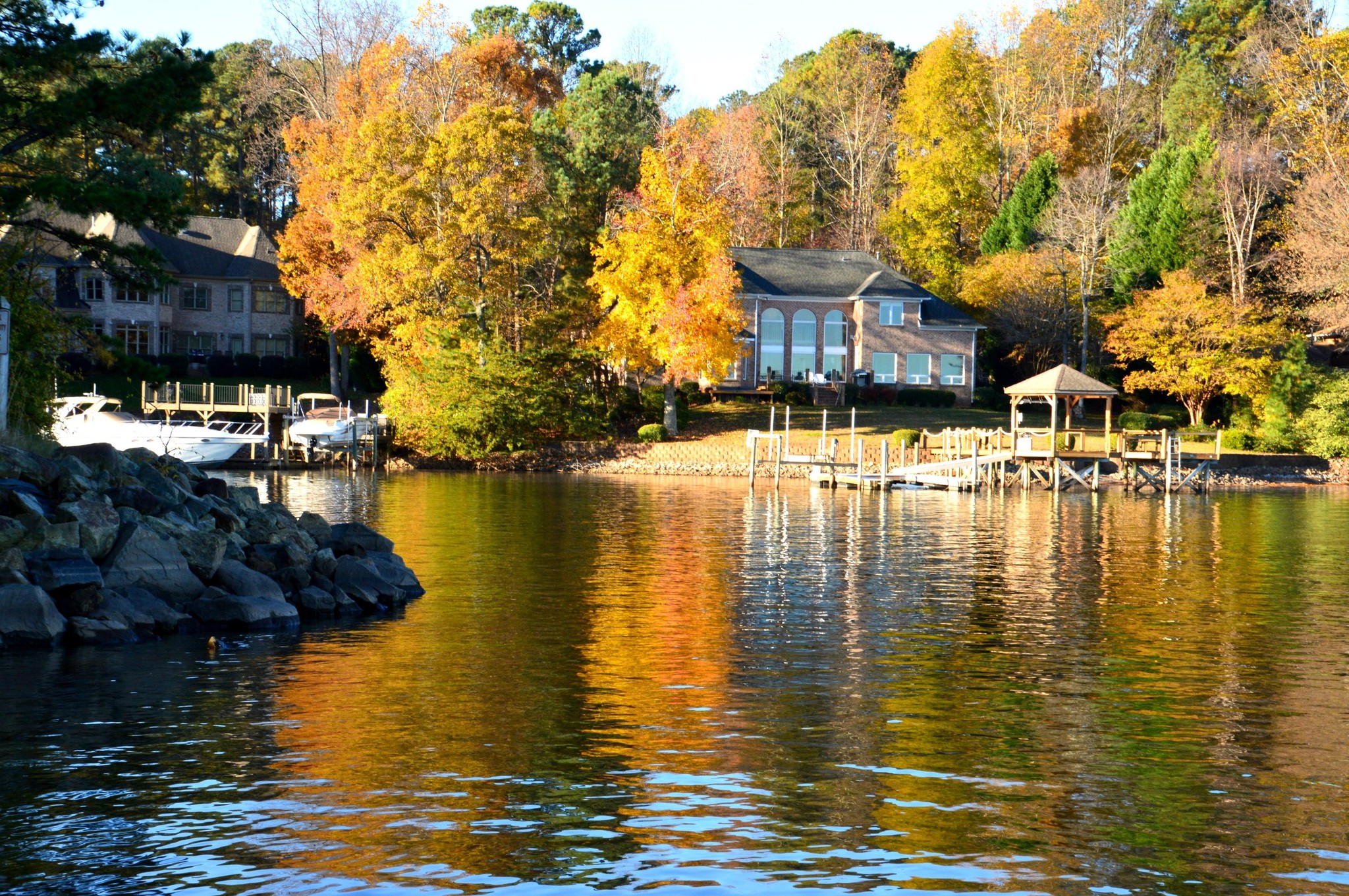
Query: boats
xmin=38 ymin=384 xmax=269 ymax=470
xmin=289 ymin=393 xmax=378 ymax=449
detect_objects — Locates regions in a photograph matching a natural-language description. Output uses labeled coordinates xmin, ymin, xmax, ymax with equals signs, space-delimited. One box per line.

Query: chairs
xmin=804 ymin=372 xmax=816 ymax=386
xmin=793 ymin=371 xmax=804 ymax=382
xmin=814 ymin=373 xmax=827 ymax=387
xmin=766 ymin=370 xmax=776 ymax=380
